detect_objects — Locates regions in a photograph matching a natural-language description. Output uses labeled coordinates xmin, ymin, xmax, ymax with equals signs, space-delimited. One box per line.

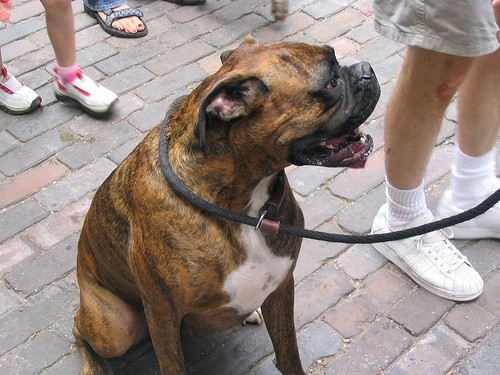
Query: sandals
xmin=83 ymin=0 xmax=148 ymax=38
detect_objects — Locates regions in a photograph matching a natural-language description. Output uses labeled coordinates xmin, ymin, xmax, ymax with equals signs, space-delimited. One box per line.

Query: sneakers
xmin=370 ymin=205 xmax=484 ymax=301
xmin=437 ymin=192 xmax=500 ymax=239
xmin=53 ymin=67 xmax=119 ymax=116
xmin=0 ymin=67 xmax=42 ymax=114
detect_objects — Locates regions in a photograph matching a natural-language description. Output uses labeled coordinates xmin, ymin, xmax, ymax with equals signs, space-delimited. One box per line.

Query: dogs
xmin=71 ymin=33 xmax=382 ymax=375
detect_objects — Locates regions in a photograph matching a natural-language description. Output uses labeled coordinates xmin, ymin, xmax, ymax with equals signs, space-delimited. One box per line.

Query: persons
xmin=0 ymin=0 xmax=120 ymax=119
xmin=367 ymin=0 xmax=500 ymax=300
xmin=82 ymin=0 xmax=150 ymax=39
